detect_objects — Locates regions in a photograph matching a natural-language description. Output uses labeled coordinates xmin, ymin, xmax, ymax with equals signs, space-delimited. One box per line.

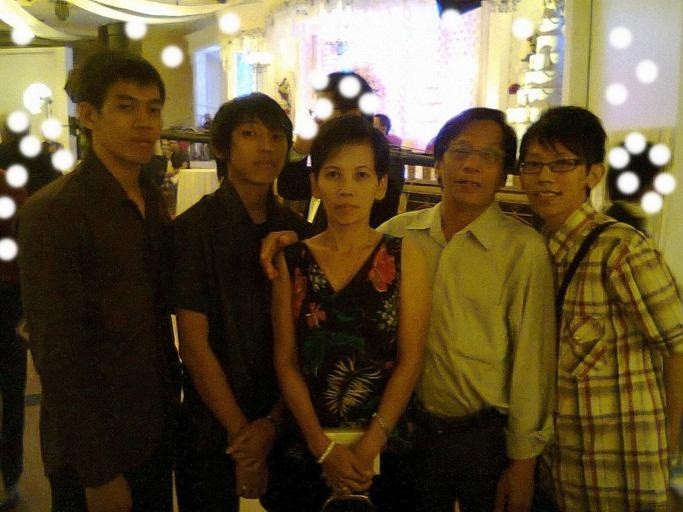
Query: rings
xmin=340 ymin=488 xmax=347 ymax=493
xmin=241 ymin=486 xmax=252 ymax=492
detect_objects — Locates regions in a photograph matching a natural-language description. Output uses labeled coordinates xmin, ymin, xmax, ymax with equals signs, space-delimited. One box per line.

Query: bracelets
xmin=313 ymin=439 xmax=337 ymax=467
xmin=265 ymin=413 xmax=280 ymax=437
xmin=371 ymin=413 xmax=392 ymax=438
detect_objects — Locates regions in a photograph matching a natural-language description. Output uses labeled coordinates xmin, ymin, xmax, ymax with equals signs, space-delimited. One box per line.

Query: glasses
xmin=519 ymin=157 xmax=589 ymax=174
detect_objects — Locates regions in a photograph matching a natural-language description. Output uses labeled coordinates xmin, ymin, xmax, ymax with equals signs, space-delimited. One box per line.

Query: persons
xmin=258 ymin=108 xmax=557 ymax=509
xmin=521 ymin=102 xmax=683 ymax=512
xmin=20 ymin=50 xmax=181 ymax=508
xmin=161 ymin=93 xmax=311 ymax=512
xmin=168 ymin=138 xmax=191 ymax=171
xmin=374 ymin=113 xmax=402 ymax=147
xmin=276 ymin=113 xmax=431 ymax=512
